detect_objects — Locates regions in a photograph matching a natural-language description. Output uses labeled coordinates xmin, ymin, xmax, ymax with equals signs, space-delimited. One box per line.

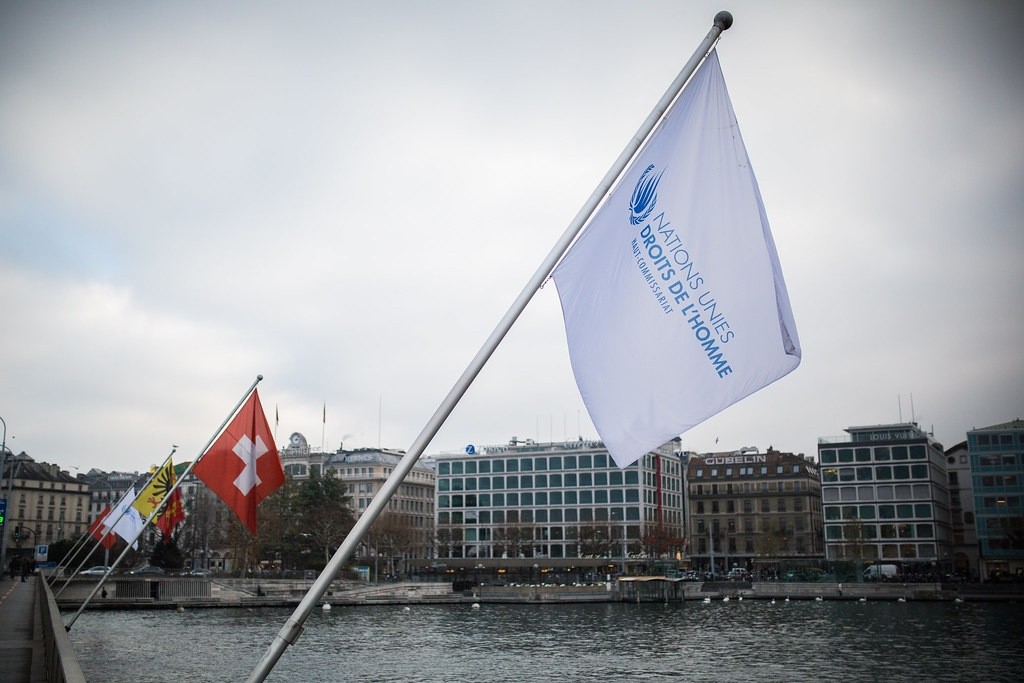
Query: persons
xmin=101 ymin=587 xmax=108 ymax=598
xmin=748 ymin=575 xmax=754 ymax=589
xmin=2 ymin=556 xmax=36 ymax=583
xmin=257 ymin=585 xmax=261 ymax=596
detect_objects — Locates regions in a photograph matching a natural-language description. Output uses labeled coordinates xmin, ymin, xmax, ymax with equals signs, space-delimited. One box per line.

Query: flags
xmin=551 ymin=48 xmax=801 ymax=470
xmin=192 ymin=388 xmax=286 ymax=536
xmin=103 ymin=487 xmax=143 ymax=551
xmin=132 ymin=459 xmax=185 ymax=544
xmin=88 ymin=505 xmax=117 ymax=550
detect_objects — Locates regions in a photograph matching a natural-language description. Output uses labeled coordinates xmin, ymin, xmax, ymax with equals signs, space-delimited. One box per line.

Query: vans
xmin=862 ymin=565 xmax=898 ymax=583
xmin=724 ymin=568 xmax=749 ymax=582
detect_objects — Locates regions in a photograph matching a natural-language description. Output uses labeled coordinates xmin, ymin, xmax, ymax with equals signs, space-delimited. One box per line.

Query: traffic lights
xmin=14 ymin=526 xmax=19 ymax=535
xmin=0 ymin=517 xmax=3 ymax=523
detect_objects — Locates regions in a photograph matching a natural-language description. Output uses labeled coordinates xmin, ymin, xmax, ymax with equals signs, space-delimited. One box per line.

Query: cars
xmin=79 ymin=566 xmax=113 ymax=576
xmin=181 ymin=569 xmax=212 ymax=577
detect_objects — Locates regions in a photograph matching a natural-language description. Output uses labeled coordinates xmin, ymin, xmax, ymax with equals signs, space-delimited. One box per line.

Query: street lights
xmin=612 ymin=510 xmax=620 ymax=574
xmin=533 ymin=564 xmax=539 ymax=598
xmin=78 ymin=474 xmax=113 ymax=578
xmin=475 ymin=564 xmax=485 ymax=598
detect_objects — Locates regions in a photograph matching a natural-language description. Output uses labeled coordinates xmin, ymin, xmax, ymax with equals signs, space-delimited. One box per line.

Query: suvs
xmin=124 ymin=565 xmax=165 ymax=577
